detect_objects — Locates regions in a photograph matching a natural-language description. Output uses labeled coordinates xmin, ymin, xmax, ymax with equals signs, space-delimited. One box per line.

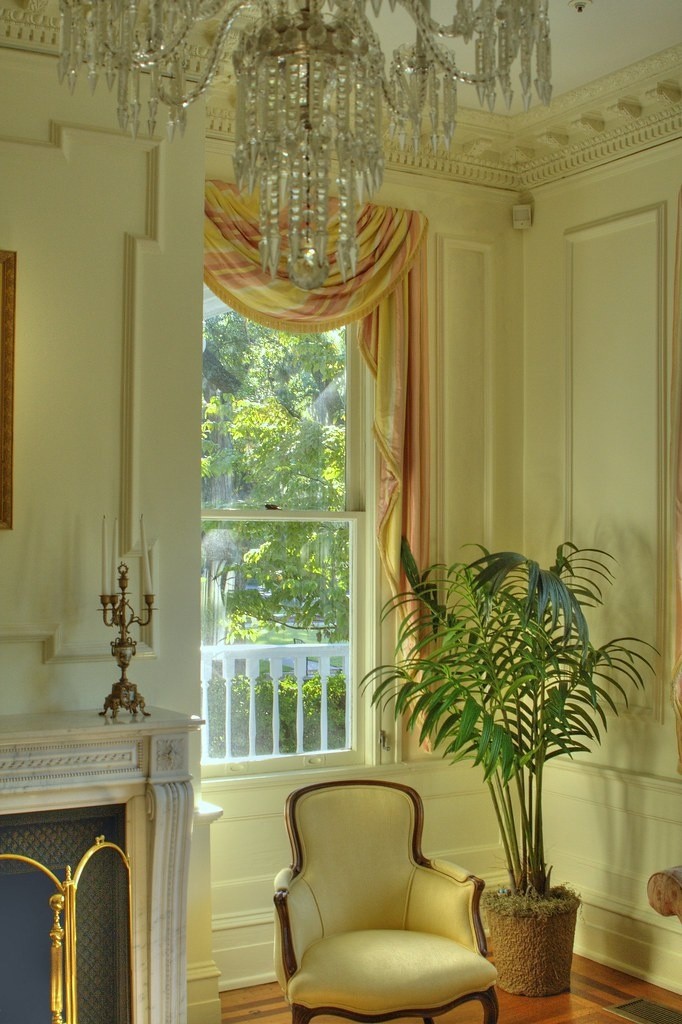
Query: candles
xmin=100 ymin=512 xmax=155 ymax=595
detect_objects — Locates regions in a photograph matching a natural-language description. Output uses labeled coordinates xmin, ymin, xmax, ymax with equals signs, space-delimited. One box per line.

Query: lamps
xmin=59 ymin=1 xmax=558 ymax=291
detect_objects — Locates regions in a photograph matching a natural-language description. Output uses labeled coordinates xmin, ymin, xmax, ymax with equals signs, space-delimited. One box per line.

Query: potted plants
xmin=359 ymin=535 xmax=665 ymax=997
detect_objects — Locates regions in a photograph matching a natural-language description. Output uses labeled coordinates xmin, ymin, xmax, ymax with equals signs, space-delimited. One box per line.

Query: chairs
xmin=274 ymin=778 xmax=499 ymax=1024
